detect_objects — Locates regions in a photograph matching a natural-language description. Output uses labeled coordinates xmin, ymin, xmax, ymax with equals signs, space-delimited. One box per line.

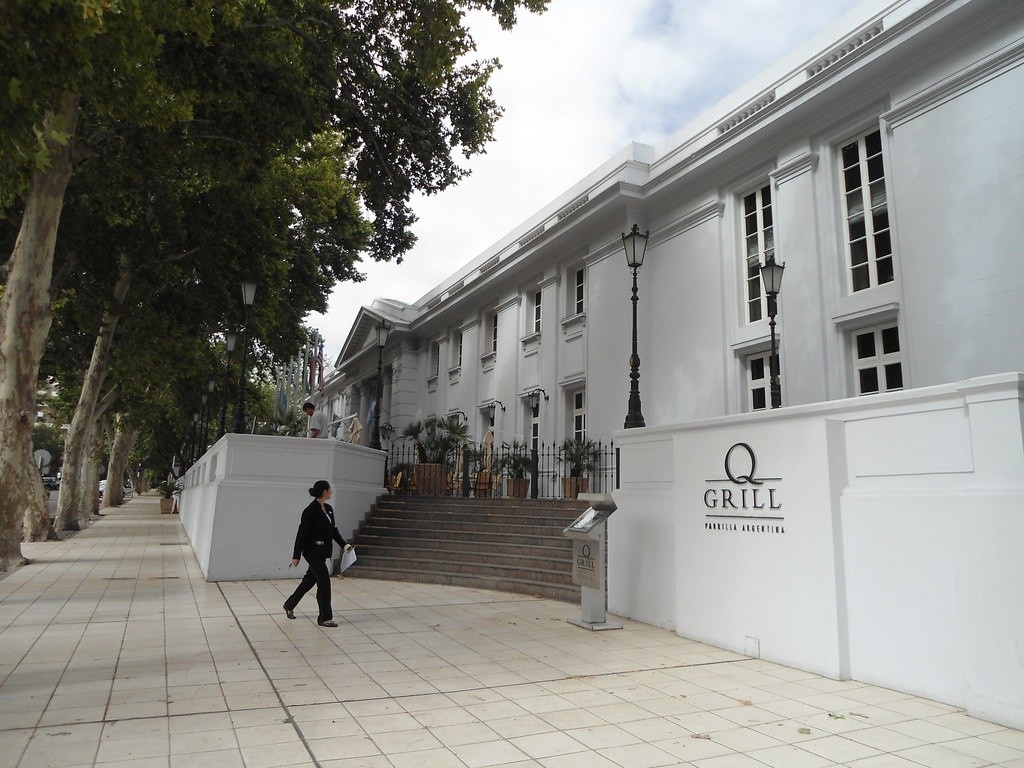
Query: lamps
xmin=488 ymin=400 xmax=506 ymax=420
xmin=453 ymin=411 xmax=468 ymax=426
xmin=425 ymin=417 xmax=440 ymax=435
xmin=527 ymin=387 xmax=550 ymax=408
xmin=379 ymin=422 xmax=395 ymax=440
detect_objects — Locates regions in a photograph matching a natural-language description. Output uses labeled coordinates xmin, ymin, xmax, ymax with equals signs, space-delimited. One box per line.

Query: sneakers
xmin=283 ymin=605 xmax=296 ymax=619
xmin=318 ymin=619 xmax=338 ymax=627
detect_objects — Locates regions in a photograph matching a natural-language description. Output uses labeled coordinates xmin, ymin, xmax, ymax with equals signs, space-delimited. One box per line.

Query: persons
xmin=283 ymin=480 xmax=353 ymax=627
xmin=302 ymin=402 xmax=330 ymax=440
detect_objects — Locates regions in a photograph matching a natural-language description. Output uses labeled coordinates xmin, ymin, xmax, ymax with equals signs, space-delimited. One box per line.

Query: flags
xmin=331 ymin=413 xmax=341 ymax=438
xmin=316 ymin=340 xmax=325 ymax=397
xmin=273 ymin=350 xmax=307 ymax=417
xmin=309 ymin=342 xmax=315 ymax=396
xmin=366 ymin=395 xmax=378 ymax=425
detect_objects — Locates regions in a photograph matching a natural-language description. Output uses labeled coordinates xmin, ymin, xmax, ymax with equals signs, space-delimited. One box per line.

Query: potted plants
xmin=463 ymin=440 xmax=502 ymax=495
xmin=501 ymin=440 xmax=535 ymax=500
xmin=404 ymin=415 xmax=467 ymax=498
xmin=559 ymin=439 xmax=601 ymax=498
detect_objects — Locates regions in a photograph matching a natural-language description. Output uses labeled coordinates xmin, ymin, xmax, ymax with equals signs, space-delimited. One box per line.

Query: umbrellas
xmin=347 ymin=416 xmax=364 ymax=445
xmin=454 ymin=435 xmax=468 ymax=473
xmin=414 ymin=443 xmax=425 ymax=464
xmin=481 ymin=428 xmax=494 ymax=472
